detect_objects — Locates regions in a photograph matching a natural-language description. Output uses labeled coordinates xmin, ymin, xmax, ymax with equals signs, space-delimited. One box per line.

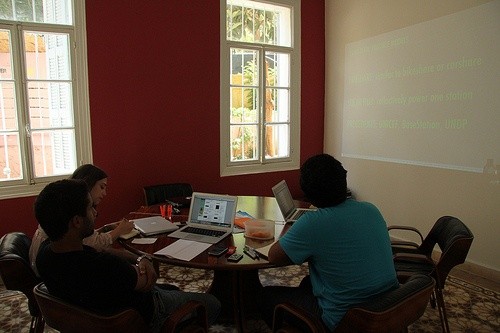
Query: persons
xmin=34 ymin=179 xmax=222 ymax=333
xmin=259 ymin=153 xmax=400 ymax=333
xmin=29 ymin=164 xmax=134 ymax=279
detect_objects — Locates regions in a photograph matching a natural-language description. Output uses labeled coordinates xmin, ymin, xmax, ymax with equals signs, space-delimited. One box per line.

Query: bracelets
xmin=103 ymin=225 xmax=113 ymax=232
xmin=136 ymin=254 xmax=152 ymax=268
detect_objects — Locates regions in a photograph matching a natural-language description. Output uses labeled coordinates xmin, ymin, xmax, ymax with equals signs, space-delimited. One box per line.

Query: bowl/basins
xmin=243 ymin=219 xmax=275 ymax=239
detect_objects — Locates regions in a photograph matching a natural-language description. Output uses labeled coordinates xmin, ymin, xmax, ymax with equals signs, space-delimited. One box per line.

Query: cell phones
xmin=207 ymin=246 xmax=227 ymax=256
xmin=227 ymin=253 xmax=243 ymax=261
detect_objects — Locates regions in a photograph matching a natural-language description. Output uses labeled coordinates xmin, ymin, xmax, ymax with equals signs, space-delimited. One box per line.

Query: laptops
xmin=129 ymin=216 xmax=179 ymax=236
xmin=168 ymin=193 xmax=238 ymax=244
xmin=271 ymin=180 xmax=318 ymax=224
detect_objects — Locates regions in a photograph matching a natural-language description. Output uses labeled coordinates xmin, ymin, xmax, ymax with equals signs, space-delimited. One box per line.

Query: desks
xmin=118 ymin=196 xmax=315 ymax=333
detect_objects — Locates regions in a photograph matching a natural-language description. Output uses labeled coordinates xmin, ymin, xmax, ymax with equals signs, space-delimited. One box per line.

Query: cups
xmin=160 ymin=204 xmax=172 ymax=221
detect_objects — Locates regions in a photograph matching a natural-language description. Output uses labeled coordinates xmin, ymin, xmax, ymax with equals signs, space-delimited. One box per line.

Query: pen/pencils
xmin=122 ymin=217 xmax=137 ymax=230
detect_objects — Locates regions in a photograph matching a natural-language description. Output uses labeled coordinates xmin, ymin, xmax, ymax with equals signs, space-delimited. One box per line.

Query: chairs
xmin=388 ymin=215 xmax=474 ymax=333
xmin=142 ymin=182 xmax=192 ymax=206
xmin=0 ymin=231 xmax=42 ymax=333
xmin=270 ymin=273 xmax=436 ymax=333
xmin=33 ymin=281 xmax=209 ymax=333
